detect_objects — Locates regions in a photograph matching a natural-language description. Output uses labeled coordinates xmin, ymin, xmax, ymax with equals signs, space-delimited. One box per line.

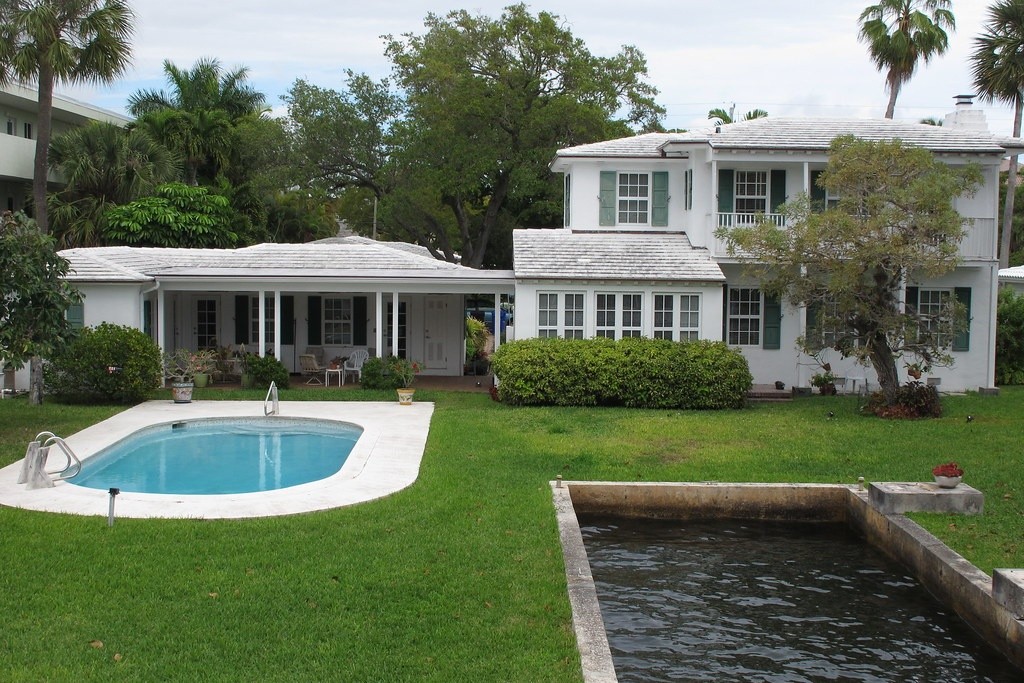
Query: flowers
xmin=190 ymin=351 xmax=222 ymax=383
xmin=389 ymin=358 xmax=426 ymax=390
xmin=932 ymin=462 xmax=964 ymax=478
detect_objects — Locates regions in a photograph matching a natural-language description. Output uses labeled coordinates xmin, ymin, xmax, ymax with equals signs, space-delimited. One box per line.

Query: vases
xmin=933 ymin=475 xmax=963 ymax=488
xmin=192 ymin=373 xmax=209 ymax=388
xmin=396 ymin=388 xmax=415 ymax=405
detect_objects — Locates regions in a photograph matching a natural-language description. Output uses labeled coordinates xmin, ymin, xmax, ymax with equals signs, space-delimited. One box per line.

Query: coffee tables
xmin=325 ymin=369 xmax=344 ymax=387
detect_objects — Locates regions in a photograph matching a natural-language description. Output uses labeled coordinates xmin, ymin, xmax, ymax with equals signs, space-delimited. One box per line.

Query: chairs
xmin=344 ymin=350 xmax=369 ymax=384
xmin=299 ymin=346 xmax=325 ymax=385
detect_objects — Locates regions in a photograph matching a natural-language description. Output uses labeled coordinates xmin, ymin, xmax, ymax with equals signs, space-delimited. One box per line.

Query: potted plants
xmin=158 ymin=348 xmax=219 ymax=403
xmin=240 ymin=352 xmax=289 ymax=388
xmin=329 ymin=356 xmax=350 ymax=369
xmin=775 ymin=380 xmax=785 ymax=390
xmin=221 ymin=343 xmax=248 ymax=360
xmin=466 ymin=316 xmax=490 ymax=376
xmin=810 ymin=372 xmax=838 ymax=396
xmin=904 ymin=361 xmax=932 ymax=380
